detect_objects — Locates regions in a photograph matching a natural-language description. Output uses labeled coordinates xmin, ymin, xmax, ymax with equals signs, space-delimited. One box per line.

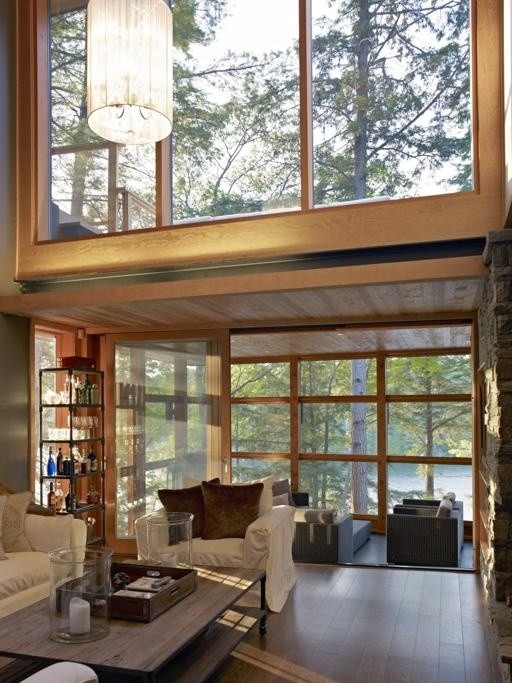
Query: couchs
xmin=271 ymin=476 xmax=372 ymax=563
xmin=0 ymin=491 xmax=88 ymax=619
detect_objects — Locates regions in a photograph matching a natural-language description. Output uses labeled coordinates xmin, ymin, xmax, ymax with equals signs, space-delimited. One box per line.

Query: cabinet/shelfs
xmin=40 ymin=369 xmax=104 ymax=545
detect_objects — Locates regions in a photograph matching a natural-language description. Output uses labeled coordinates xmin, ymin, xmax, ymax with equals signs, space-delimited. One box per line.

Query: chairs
xmin=134 ymin=475 xmax=300 ymax=614
xmin=20 ymin=662 xmax=98 ymax=683
xmin=386 ymin=492 xmax=463 ymax=567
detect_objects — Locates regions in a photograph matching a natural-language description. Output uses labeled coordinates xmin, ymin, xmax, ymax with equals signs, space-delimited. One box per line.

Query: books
xmin=114 ymin=589 xmax=156 ymax=600
xmin=127 ymin=575 xmax=180 ymax=593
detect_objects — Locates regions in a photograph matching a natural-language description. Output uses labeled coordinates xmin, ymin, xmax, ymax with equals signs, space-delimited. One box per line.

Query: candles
xmin=69 ymin=597 xmax=91 ymax=635
xmin=160 ymin=552 xmax=178 ymax=568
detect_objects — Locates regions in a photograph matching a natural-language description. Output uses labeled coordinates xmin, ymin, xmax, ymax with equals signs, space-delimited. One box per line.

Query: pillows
xmin=273 ymin=479 xmax=309 ymax=506
xmin=435 ymin=492 xmax=455 ymax=517
xmin=158 ymin=474 xmax=273 ymax=545
xmin=304 ymin=506 xmax=336 ymax=524
xmin=0 ymin=492 xmax=74 ymax=560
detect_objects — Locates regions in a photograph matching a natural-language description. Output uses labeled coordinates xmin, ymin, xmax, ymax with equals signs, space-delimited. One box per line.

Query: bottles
xmin=44 ymin=374 xmax=100 ymax=513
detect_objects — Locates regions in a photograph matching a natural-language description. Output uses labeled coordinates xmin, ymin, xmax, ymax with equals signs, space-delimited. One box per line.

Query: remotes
xmin=151 ymin=575 xmax=172 ymax=589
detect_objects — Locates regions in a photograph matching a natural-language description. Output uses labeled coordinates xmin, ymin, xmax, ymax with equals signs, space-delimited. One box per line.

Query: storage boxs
xmin=57 ymin=357 xmax=96 ymax=371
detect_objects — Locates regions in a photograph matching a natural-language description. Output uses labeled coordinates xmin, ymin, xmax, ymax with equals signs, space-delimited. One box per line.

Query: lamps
xmin=86 ymin=0 xmax=173 ymax=145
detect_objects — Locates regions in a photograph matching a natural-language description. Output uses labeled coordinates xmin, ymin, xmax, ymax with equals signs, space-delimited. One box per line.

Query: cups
xmin=134 ymin=511 xmax=195 ymax=568
xmin=48 ymin=545 xmax=115 ymax=644
